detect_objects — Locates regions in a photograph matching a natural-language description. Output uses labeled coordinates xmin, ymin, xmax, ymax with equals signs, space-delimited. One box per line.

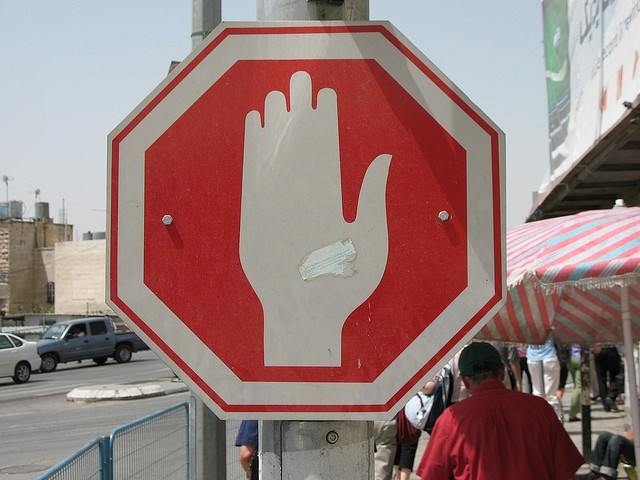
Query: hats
xmin=458 ymin=341 xmax=500 ymax=376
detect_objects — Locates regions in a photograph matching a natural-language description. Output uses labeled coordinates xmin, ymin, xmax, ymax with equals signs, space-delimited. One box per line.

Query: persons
xmin=235 ymin=420 xmax=258 ymax=479
xmin=393 ymin=407 xmax=423 ymax=480
xmin=422 ymin=343 xmax=473 ymax=414
xmin=516 ymin=343 xmax=533 ymax=395
xmin=592 ymin=342 xmax=625 ymax=413
xmin=585 ymin=426 xmax=637 ymax=480
xmin=554 ymin=343 xmax=568 ymax=400
xmin=525 ymin=342 xmax=564 ymax=426
xmin=374 ymin=413 xmax=397 ymax=479
xmin=567 ymin=343 xmax=583 ymax=423
xmin=414 ymin=343 xmax=586 ymax=479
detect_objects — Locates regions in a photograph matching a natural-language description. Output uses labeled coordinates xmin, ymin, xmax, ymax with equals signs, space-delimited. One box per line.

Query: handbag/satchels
xmin=404 ymin=391 xmax=434 ymax=431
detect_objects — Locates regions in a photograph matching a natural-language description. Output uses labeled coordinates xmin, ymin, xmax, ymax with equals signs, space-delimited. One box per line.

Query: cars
xmin=0 ymin=333 xmax=42 ymax=384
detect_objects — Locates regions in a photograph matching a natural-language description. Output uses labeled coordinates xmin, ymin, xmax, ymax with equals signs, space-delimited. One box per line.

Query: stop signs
xmin=105 ymin=20 xmax=509 ymax=421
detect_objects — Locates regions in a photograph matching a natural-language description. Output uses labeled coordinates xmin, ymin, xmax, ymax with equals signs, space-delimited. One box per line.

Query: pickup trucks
xmin=37 ymin=317 xmax=151 ymax=373
